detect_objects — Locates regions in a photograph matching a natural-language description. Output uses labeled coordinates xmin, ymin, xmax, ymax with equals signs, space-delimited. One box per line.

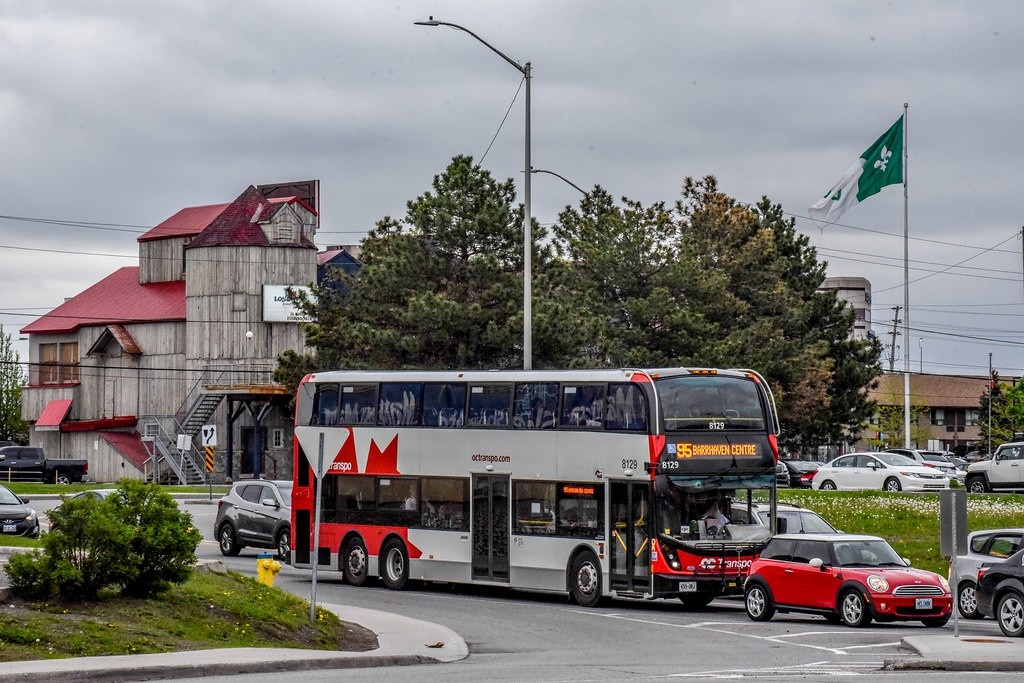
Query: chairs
xmin=837 ymin=545 xmax=855 ymax=563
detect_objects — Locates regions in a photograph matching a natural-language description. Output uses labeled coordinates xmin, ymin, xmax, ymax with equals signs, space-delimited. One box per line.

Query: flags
xmin=808 ymin=114 xmax=903 ymax=231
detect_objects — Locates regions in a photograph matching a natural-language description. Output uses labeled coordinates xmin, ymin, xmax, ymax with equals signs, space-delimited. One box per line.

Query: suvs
xmin=213 ymin=479 xmax=294 ymax=561
xmin=964 ymin=442 xmax=1024 ymax=495
xmin=883 ymin=448 xmax=958 ymax=484
xmin=727 ymin=499 xmax=846 ymax=542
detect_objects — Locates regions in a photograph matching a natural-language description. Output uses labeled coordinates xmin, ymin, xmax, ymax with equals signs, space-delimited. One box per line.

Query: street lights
xmin=412 ymin=15 xmax=534 ymax=373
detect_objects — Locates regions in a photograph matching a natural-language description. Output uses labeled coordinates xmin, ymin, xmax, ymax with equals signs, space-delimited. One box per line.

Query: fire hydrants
xmin=257 ymin=552 xmax=282 ymax=590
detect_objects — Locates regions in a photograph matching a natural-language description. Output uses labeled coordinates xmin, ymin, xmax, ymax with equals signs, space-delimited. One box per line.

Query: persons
xmin=701 ymin=501 xmax=732 ymax=532
xmin=403 ymin=482 xmax=454 ymax=530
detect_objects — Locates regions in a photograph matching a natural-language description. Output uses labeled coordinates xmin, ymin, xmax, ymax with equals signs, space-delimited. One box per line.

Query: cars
xmin=743 ymin=532 xmax=954 ymax=629
xmin=938 ymin=449 xmax=989 ymax=473
xmin=811 ymin=452 xmax=952 ymax=493
xmin=975 ymin=547 xmax=1024 ymax=637
xmin=775 ymin=459 xmax=825 ymax=489
xmin=948 ymin=528 xmax=1024 ymax=620
xmin=49 ymin=488 xmax=148 ymax=534
xmin=0 ymin=484 xmax=40 ymax=539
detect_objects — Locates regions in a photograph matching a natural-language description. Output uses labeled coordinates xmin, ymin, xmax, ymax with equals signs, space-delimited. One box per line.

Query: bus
xmin=290 ymin=368 xmax=782 ymax=608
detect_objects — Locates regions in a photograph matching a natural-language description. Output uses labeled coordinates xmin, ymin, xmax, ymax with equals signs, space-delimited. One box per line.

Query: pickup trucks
xmin=0 ymin=445 xmax=89 ymax=486
